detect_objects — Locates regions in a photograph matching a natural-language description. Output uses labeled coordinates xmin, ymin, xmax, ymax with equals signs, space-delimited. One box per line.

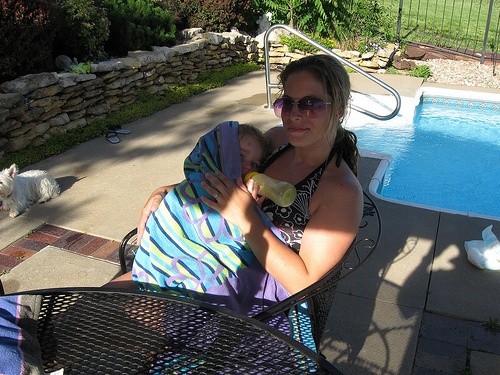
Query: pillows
xmin=0 ymin=295 xmax=46 ymax=375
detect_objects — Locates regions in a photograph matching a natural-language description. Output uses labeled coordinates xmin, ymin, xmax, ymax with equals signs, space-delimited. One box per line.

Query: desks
xmin=0 ymin=287 xmax=344 ymax=375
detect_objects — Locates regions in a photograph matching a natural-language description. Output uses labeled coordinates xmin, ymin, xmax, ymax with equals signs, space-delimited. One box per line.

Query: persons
xmin=238 ymin=124 xmax=267 ymax=210
xmin=38 ymin=54 xmax=364 ymax=374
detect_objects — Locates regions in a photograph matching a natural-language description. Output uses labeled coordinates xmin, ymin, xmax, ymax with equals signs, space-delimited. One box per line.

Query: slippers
xmin=104 ymin=132 xmax=120 ymax=144
xmin=104 ymin=125 xmax=131 ymax=134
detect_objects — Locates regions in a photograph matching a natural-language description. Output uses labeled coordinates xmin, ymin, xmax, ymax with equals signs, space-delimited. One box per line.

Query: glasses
xmin=273 ymin=96 xmax=333 ymax=118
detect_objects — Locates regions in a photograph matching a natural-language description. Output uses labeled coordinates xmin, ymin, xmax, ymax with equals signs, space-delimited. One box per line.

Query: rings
xmin=215 ymin=193 xmax=220 ymax=198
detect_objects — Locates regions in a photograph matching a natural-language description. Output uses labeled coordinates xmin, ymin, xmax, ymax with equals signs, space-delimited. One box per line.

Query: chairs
xmin=120 ymin=192 xmax=381 ymax=362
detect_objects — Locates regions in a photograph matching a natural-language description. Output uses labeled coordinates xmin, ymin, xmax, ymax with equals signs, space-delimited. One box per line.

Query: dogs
xmin=1 ymin=164 xmax=61 ymax=218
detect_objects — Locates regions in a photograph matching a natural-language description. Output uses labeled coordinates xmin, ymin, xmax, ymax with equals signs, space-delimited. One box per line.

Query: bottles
xmin=244 ymin=171 xmax=297 ymax=208
